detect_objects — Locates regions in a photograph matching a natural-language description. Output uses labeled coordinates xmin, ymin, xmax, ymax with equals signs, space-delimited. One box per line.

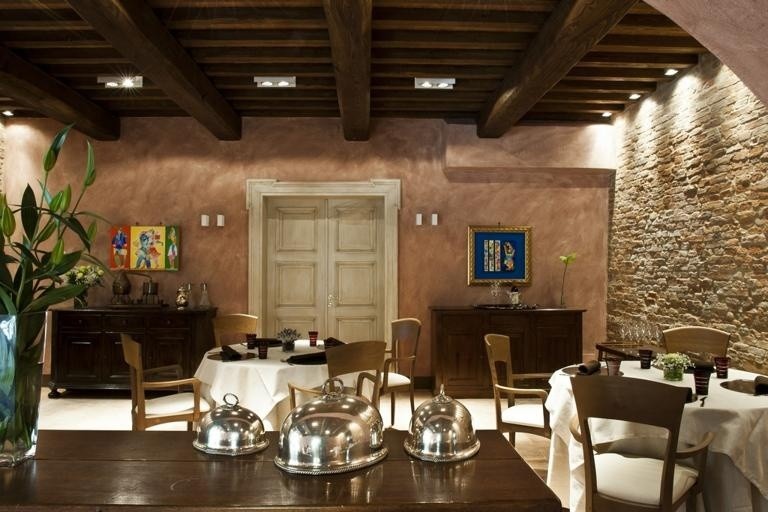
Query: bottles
xmin=509 ymin=286 xmax=518 ymax=305
xmin=186 ymin=282 xmax=211 ymax=309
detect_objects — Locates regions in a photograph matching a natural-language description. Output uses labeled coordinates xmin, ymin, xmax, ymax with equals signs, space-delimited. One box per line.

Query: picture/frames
xmin=466 ymin=225 xmax=533 ymax=287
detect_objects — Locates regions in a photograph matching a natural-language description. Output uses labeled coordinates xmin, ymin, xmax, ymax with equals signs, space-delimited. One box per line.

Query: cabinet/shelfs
xmin=49 ymin=307 xmax=217 ymax=400
xmin=428 ymin=303 xmax=587 ymax=399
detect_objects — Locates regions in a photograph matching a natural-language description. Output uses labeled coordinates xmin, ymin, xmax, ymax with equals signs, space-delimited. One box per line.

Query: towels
xmin=577 ymin=360 xmax=601 ymax=375
xmin=688 ymin=357 xmax=715 ymax=372
xmin=220 ymin=337 xmax=345 ymax=365
xmin=754 ymin=376 xmax=768 ymax=395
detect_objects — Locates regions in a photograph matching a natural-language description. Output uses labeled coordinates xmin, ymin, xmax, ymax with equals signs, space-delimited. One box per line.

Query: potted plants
xmin=277 ymin=328 xmax=301 ymax=352
xmin=559 ymin=253 xmax=576 ymax=308
xmin=655 ymin=352 xmax=696 ymax=380
xmin=0 ymin=122 xmax=116 ymax=470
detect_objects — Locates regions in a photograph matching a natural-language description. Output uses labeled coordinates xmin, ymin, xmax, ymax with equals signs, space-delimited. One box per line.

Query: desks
xmin=596 ymin=340 xmax=666 ymax=376
xmin=0 ymin=429 xmax=561 ymax=512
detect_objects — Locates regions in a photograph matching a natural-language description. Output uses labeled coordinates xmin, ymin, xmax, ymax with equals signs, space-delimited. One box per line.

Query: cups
xmin=713 ymin=356 xmax=730 ymax=378
xmin=308 ymin=331 xmax=318 ymax=346
xmin=639 ymin=349 xmax=652 ymax=369
xmin=693 ymin=366 xmax=713 ymax=395
xmin=245 ymin=334 xmax=257 ymax=349
xmin=604 ymin=357 xmax=622 ymax=377
xmin=257 ymin=343 xmax=267 ymax=359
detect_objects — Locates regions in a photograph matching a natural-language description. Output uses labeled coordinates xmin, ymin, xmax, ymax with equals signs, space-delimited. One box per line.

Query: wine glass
xmin=617 ymin=319 xmax=663 ymax=348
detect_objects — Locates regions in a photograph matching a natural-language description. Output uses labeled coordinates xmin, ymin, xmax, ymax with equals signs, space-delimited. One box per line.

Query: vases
xmin=73 ymin=288 xmax=89 ymax=309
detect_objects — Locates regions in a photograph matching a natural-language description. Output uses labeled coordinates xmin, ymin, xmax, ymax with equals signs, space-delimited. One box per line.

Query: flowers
xmin=64 ymin=265 xmax=104 ymax=308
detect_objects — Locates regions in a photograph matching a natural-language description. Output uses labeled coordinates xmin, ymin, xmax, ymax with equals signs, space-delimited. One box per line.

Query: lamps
xmin=254 ymin=77 xmax=296 ymax=88
xmin=97 ymin=77 xmax=143 ymax=88
xmin=415 ymin=79 xmax=455 ymax=90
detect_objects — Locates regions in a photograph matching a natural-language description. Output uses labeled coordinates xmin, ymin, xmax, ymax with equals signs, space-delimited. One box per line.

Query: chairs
xmin=379 ymin=317 xmax=421 ymax=426
xmin=484 ymin=333 xmax=551 ymax=448
xmin=288 ymin=340 xmax=387 ymax=412
xmin=212 ymin=313 xmax=259 ymax=347
xmin=119 ymin=333 xmax=209 ymax=432
xmin=570 ymin=374 xmax=715 ymax=512
xmin=662 ymin=325 xmax=730 ymax=357
